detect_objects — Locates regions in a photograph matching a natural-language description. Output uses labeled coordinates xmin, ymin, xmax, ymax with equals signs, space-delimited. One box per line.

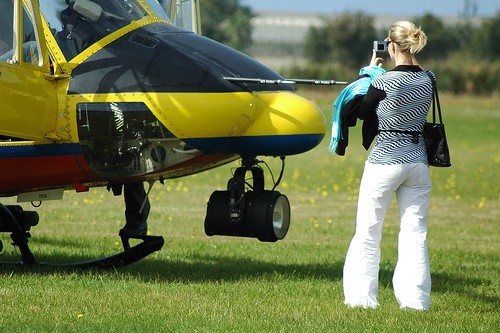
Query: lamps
xmin=204 ymin=157 xmax=291 ymax=243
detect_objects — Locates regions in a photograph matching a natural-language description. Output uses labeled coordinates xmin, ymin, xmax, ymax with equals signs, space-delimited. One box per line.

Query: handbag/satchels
xmin=424 ymin=70 xmax=451 ymax=167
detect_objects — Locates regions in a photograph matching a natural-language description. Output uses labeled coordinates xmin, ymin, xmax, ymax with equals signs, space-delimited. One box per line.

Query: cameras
xmin=373 ymin=40 xmax=388 ymax=52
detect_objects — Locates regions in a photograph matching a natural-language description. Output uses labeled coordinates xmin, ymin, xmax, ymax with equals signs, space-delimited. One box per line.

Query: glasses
xmin=384 ymin=37 xmax=401 ymax=47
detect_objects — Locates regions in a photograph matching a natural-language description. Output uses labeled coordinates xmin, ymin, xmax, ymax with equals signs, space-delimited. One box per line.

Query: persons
xmin=342 ymin=21 xmax=437 ymax=311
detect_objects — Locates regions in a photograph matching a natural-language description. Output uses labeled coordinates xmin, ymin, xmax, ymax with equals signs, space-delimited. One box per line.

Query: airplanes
xmin=1 ymin=1 xmax=351 ymax=273
